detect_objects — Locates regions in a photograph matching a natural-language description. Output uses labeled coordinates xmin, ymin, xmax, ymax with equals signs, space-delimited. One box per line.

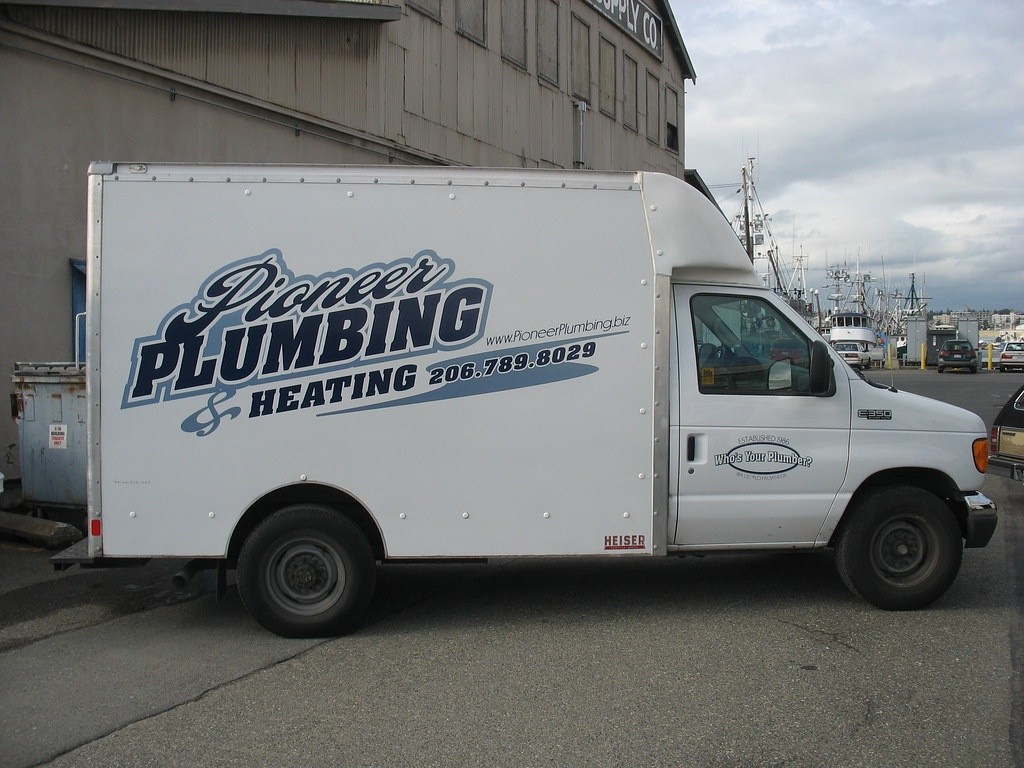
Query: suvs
xmin=999 ymin=341 xmax=1024 ymax=373
xmin=935 ymin=339 xmax=977 ymax=374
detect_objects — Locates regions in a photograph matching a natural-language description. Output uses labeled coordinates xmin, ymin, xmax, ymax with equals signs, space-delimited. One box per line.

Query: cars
xmin=832 ymin=342 xmax=872 ymax=371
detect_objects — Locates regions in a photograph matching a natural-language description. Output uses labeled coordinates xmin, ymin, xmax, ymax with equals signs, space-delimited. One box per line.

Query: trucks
xmin=87 ymin=159 xmax=995 ymax=642
